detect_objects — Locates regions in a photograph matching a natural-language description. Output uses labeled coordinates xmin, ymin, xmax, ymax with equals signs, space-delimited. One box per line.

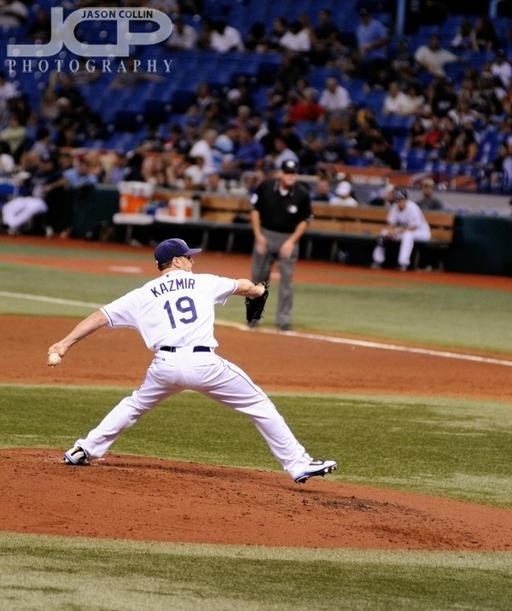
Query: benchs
xmin=148 ymin=191 xmax=456 ymax=274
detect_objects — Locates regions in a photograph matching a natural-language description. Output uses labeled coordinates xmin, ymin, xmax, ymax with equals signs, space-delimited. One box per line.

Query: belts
xmin=160 ymin=345 xmax=213 ymax=352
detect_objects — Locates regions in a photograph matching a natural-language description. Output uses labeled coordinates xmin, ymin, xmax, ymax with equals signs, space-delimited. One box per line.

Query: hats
xmin=154 ymin=238 xmax=203 ymax=262
xmin=335 ymin=181 xmax=352 ymax=197
xmin=282 ymin=159 xmax=298 ymax=175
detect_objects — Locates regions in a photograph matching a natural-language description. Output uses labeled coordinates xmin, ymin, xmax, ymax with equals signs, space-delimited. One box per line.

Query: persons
xmin=46 ymin=239 xmax=338 ymax=482
xmin=373 ymin=186 xmax=432 ymax=269
xmin=240 ymin=160 xmax=315 ymax=331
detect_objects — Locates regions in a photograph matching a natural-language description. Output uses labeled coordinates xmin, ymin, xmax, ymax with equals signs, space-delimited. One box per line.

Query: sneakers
xmin=288 ymin=459 xmax=338 ymax=484
xmin=62 ymin=446 xmax=88 ymax=465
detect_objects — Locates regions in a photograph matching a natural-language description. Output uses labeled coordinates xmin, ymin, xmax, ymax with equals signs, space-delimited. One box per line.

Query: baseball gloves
xmin=244 ymin=279 xmax=270 ymax=327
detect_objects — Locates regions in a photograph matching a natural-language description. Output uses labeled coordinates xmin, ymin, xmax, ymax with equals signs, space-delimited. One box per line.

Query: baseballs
xmin=48 ymin=352 xmax=61 ymax=365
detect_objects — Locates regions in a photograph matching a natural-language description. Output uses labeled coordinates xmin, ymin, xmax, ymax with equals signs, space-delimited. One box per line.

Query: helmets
xmin=394 ymin=188 xmax=408 ymax=201
